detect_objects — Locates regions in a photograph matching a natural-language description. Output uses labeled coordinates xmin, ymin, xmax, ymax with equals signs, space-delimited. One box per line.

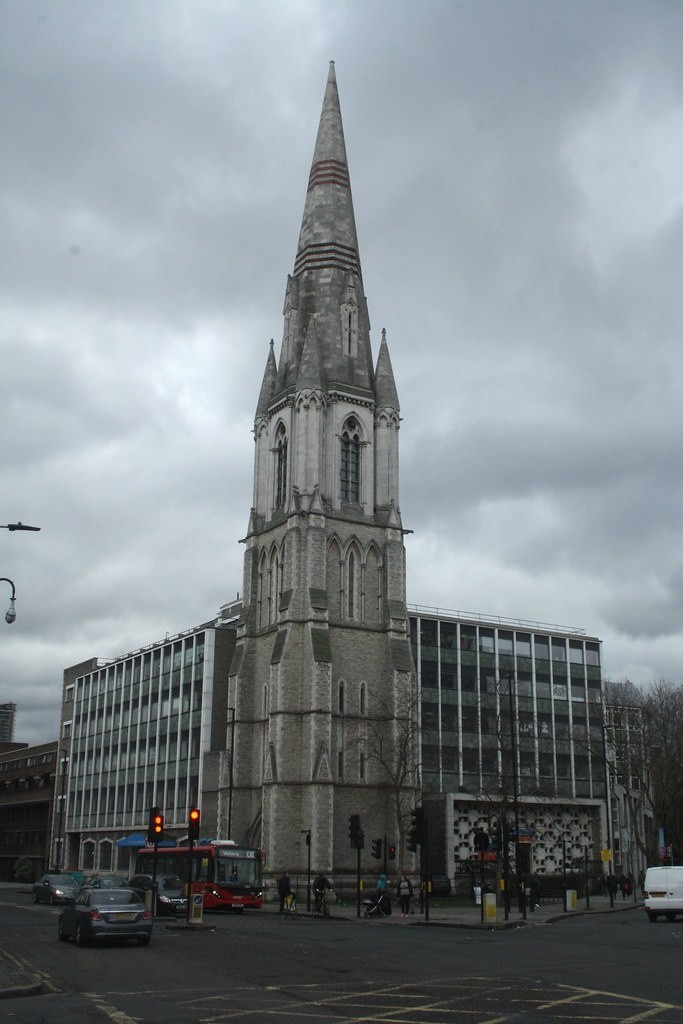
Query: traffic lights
xmin=405 ymin=828 xmax=417 ymax=852
xmin=371 ymin=839 xmax=381 ymax=859
xmin=150 ymin=815 xmax=164 ymax=842
xmin=411 ymin=807 xmax=423 ymax=830
xmin=306 ymin=834 xmax=310 ymax=845
xmin=389 ymin=846 xmax=395 ymax=859
xmin=348 ymin=815 xmax=359 ymax=838
xmin=189 ymin=809 xmax=200 ymax=840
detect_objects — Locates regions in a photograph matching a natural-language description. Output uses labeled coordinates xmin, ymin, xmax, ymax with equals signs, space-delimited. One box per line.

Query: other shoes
xmin=405 ymin=914 xmax=408 ymax=917
xmin=317 ymin=909 xmax=322 ymax=913
xmin=534 ymin=904 xmax=540 ymax=909
xmin=401 ymin=913 xmax=405 ymax=917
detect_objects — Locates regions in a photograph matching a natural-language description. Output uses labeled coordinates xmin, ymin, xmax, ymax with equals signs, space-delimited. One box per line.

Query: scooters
xmin=472 ymin=880 xmax=492 ymax=903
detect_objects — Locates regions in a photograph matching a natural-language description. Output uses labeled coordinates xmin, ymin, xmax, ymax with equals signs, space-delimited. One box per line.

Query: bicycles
xmin=282 ymin=893 xmax=298 ymax=919
xmin=312 ymin=889 xmax=332 ymax=919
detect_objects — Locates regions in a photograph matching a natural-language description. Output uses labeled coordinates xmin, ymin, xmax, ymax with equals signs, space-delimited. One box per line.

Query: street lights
xmin=504 ymin=673 xmax=523 ymax=914
xmin=602 ymin=723 xmax=621 ymax=907
xmin=227 ymin=707 xmax=235 ymax=839
xmin=56 ymin=748 xmax=68 ymax=874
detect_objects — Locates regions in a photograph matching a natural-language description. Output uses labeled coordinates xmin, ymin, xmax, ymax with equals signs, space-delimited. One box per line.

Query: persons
xmin=278 ymin=871 xmax=295 ymax=912
xmin=312 ymin=871 xmax=332 ymax=913
xmin=375 ymin=867 xmax=386 ymax=898
xmin=639 ymin=870 xmax=646 ymax=896
xmin=605 ymin=872 xmax=632 ymax=900
xmin=396 ymin=875 xmax=414 ymax=918
xmin=533 ymin=873 xmax=543 ymax=908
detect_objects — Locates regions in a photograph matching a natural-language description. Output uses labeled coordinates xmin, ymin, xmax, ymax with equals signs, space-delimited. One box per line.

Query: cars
xmin=58 ymin=888 xmax=153 ymax=948
xmin=32 ymin=875 xmax=81 ymax=905
xmin=82 ymin=877 xmax=130 ymax=888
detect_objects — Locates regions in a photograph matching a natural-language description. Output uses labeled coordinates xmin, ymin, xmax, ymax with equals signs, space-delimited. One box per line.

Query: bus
xmin=134 ymin=840 xmax=266 ymax=914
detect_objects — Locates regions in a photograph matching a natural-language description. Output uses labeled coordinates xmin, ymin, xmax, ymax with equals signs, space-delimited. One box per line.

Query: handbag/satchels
xmin=623 ymin=883 xmax=629 ymax=891
xmin=410 ymin=900 xmax=416 ymax=914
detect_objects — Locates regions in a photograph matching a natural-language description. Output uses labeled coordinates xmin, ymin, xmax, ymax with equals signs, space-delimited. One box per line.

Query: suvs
xmin=129 ymin=874 xmax=189 ymax=917
xmin=425 ymin=873 xmax=451 ymax=896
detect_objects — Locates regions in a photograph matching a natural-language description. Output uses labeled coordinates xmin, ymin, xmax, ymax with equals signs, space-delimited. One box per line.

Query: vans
xmin=644 ymin=866 xmax=683 ymax=922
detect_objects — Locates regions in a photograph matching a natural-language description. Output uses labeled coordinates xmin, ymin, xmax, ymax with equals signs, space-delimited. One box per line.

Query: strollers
xmin=363 ymin=894 xmax=388 ymax=917
